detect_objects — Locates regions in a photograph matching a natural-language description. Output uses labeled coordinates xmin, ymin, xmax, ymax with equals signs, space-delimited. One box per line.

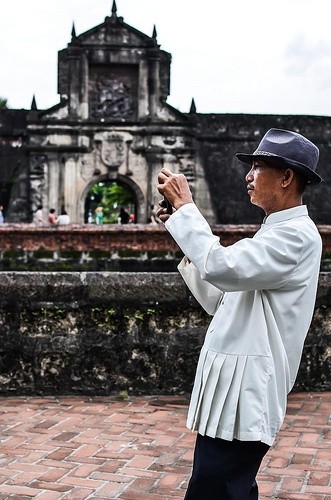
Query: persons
xmin=158 ymin=128 xmax=324 ymax=500
xmin=0 ymin=205 xmax=4 ymax=223
xmin=48 ymin=208 xmax=58 ymax=226
xmin=94 ymin=206 xmax=104 ymax=225
xmin=118 ymin=207 xmax=129 ymax=225
xmin=33 ymin=204 xmax=47 ymax=227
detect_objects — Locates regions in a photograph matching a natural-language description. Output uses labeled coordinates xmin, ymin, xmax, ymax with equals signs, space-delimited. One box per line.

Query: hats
xmin=235 ymin=128 xmax=322 ymax=184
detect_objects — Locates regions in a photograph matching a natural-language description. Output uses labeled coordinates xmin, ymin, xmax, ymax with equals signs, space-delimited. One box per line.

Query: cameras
xmin=164 ymin=196 xmax=172 ymax=214
